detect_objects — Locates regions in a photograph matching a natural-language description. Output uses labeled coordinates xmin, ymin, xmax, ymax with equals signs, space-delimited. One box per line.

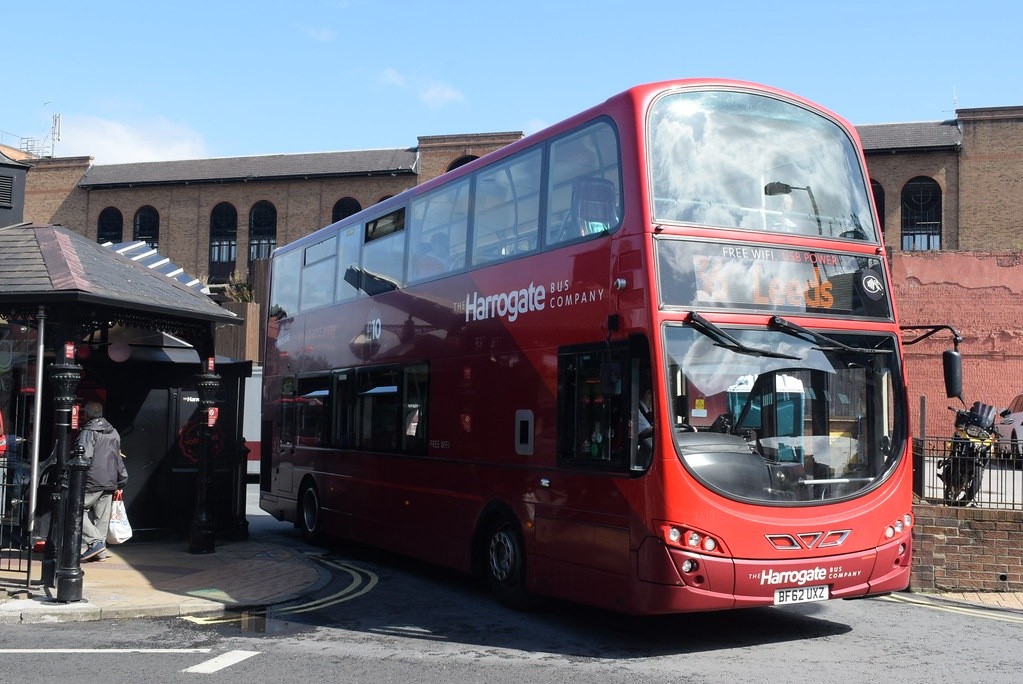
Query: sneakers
xmin=81 ymin=541 xmax=106 ymax=561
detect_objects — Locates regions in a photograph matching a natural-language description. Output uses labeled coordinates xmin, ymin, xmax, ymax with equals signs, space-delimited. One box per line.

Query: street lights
xmin=765 ymin=182 xmax=822 ymax=237
xmin=349 ymin=313 xmax=441 ymax=362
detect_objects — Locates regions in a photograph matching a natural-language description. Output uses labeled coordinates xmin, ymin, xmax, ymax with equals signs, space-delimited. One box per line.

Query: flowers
xmin=231 ymin=282 xmax=252 ymax=303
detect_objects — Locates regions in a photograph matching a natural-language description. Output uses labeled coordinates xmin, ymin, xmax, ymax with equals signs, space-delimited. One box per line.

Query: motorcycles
xmin=935 ymin=393 xmax=1005 ymax=508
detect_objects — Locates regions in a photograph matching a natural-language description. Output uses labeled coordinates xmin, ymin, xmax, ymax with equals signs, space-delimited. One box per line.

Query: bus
xmin=257 ymin=78 xmax=964 ymax=617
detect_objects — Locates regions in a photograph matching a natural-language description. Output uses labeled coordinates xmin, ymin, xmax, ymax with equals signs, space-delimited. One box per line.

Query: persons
xmin=616 ymin=377 xmax=653 ymax=458
xmin=609 ymin=365 xmax=630 ymax=413
xmin=76 ymin=402 xmax=128 ymax=560
xmin=415 ymin=233 xmax=451 ymax=279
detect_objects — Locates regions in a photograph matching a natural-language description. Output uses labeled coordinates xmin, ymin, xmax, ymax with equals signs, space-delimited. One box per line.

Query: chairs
xmin=366 ymin=178 xmax=615 ymax=296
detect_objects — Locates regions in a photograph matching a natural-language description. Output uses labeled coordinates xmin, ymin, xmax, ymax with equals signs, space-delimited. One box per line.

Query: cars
xmin=993 ymin=393 xmax=1023 ymax=470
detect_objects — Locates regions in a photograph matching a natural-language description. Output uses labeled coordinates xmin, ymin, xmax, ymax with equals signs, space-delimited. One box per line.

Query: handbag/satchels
xmin=108 ymin=490 xmax=133 ymax=545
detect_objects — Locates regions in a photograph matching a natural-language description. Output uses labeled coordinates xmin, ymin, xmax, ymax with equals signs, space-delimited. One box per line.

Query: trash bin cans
xmin=726 ymin=372 xmax=806 ymax=462
